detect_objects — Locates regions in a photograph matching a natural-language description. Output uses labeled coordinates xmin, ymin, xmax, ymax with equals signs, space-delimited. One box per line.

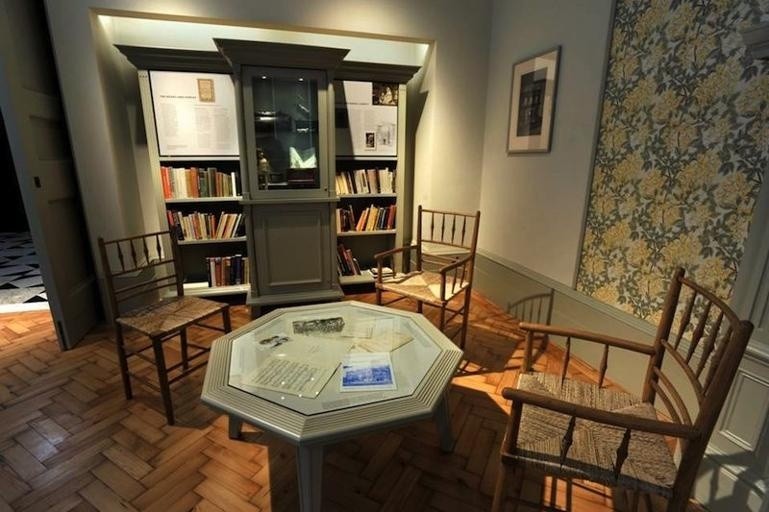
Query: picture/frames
xmin=506 ymin=46 xmax=561 ymax=155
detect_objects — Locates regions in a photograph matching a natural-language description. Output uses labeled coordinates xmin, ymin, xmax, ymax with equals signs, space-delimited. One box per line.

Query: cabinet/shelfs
xmin=334 ymin=156 xmax=402 ymax=286
xmin=235 ymin=66 xmax=337 ymax=198
xmin=159 ymin=156 xmax=251 ymax=296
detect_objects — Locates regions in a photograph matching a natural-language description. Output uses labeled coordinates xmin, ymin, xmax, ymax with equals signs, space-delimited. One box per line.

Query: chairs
xmin=374 ymin=205 xmax=482 ymax=351
xmin=490 ymin=267 xmax=754 ymax=512
xmin=97 ymin=226 xmax=233 ymax=425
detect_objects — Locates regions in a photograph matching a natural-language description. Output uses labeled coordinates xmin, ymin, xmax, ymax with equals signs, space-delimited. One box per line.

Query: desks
xmin=199 ymin=301 xmax=464 ymax=512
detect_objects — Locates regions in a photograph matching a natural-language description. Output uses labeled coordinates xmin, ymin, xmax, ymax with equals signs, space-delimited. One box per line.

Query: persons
xmin=259 ymin=335 xmax=280 ymax=346
xmin=273 ymin=338 xmax=290 ymax=349
xmin=377 ymin=91 xmax=385 ymax=102
xmin=382 ymin=85 xmax=395 ymax=105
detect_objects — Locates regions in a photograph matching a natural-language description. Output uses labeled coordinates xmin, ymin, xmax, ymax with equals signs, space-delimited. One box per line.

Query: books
xmin=159 ymin=165 xmax=250 ymax=291
xmin=339 ymin=351 xmax=398 ymax=393
xmin=358 ymin=329 xmax=413 ymax=354
xmin=240 ymin=336 xmax=356 ymax=399
xmin=340 ymin=318 xmax=377 ymax=340
xmin=336 ymin=166 xmax=396 ymax=280
